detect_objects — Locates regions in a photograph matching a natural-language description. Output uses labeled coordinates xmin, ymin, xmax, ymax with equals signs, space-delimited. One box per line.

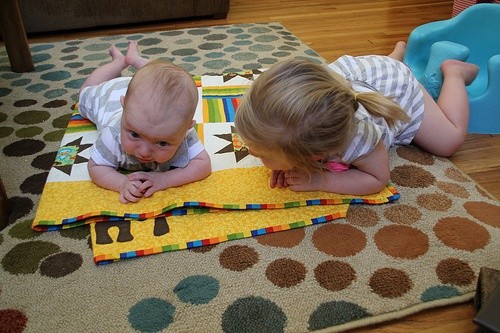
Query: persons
xmin=236 ymin=40 xmax=480 ymax=196
xmin=78 ymin=41 xmax=212 ymax=204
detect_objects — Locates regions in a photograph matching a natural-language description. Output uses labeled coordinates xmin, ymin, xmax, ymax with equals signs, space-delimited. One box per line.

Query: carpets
xmin=0 ymin=20 xmax=500 ymax=333
xmin=31 ymin=67 xmax=400 ymax=265
